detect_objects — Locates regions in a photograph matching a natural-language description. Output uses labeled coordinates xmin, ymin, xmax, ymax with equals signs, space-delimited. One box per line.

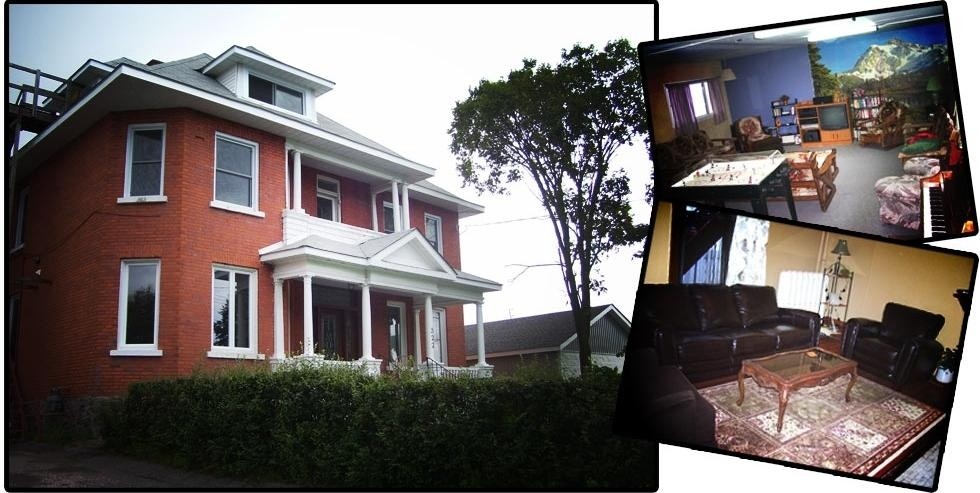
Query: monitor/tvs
xmin=818 ymin=103 xmax=849 ymax=130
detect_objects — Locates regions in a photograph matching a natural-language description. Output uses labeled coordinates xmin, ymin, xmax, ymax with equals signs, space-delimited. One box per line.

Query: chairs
xmin=836 ymin=300 xmax=945 ymax=391
xmin=613 ymin=340 xmax=717 ymax=447
xmin=855 ymin=97 xmax=962 ymax=231
xmin=728 ymin=112 xmax=785 ymax=154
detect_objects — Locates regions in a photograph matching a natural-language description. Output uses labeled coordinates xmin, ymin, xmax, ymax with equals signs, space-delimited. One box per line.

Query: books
xmin=773 ymin=104 xmax=799 ymax=126
xmin=852 ymin=87 xmax=885 ymax=131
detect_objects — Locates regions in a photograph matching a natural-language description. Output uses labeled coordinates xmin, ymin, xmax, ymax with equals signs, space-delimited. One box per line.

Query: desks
xmin=666 ymin=148 xmax=800 ymax=223
xmin=736 ymin=345 xmax=859 ymax=434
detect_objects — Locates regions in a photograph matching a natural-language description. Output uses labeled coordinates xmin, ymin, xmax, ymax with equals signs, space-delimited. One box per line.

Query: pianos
xmin=919 ymin=162 xmax=974 ymax=240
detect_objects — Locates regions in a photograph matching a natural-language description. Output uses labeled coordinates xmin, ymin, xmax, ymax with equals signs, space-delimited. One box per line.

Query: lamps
xmin=926 ymin=74 xmax=945 ymax=106
xmin=829 ymin=238 xmax=853 ymax=289
xmin=717 ymin=55 xmax=737 ymax=82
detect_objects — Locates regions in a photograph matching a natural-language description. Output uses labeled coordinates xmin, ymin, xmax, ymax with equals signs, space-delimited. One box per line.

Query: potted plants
xmin=930 ymin=343 xmax=961 ymax=384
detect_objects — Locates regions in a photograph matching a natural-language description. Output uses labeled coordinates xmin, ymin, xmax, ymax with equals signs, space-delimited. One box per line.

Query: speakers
xmin=804 ymin=131 xmax=820 ymax=142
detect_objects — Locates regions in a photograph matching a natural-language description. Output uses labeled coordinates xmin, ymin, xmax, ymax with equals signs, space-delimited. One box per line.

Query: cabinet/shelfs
xmin=769 ymin=94 xmax=799 ymax=145
xmin=820 ymin=127 xmax=853 ymax=144
xmin=792 ymin=102 xmax=822 ymax=146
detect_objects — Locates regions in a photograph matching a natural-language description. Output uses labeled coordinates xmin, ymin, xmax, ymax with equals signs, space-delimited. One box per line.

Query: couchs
xmin=652 ymin=128 xmax=738 ymax=195
xmin=719 ymin=147 xmax=840 ymax=213
xmin=635 ymin=280 xmax=823 ymax=387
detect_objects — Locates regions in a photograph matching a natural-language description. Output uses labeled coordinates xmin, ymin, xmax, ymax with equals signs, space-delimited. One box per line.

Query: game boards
xmin=672 ymin=159 xmax=783 ymax=185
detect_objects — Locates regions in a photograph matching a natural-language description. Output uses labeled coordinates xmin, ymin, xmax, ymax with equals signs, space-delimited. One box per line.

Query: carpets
xmin=693 ymin=368 xmax=948 ymax=481
